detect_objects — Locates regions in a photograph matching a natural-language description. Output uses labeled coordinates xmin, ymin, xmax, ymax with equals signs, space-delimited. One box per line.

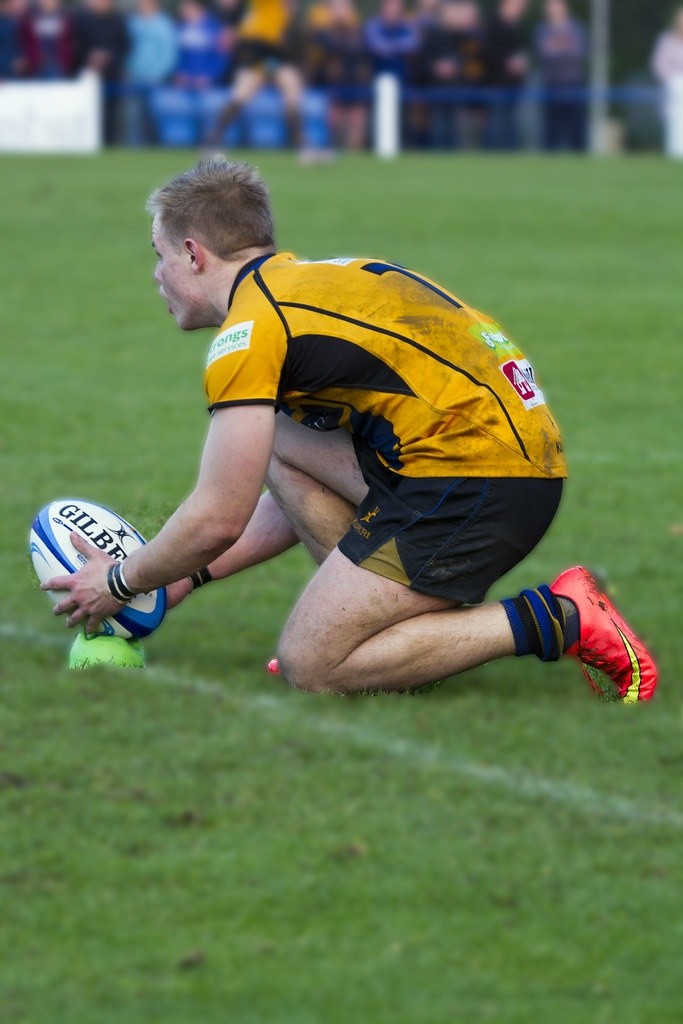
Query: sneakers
xmin=550 ymin=566 xmax=658 ymax=706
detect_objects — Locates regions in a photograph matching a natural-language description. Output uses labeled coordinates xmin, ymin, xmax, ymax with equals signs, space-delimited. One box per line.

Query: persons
xmin=0 ymin=0 xmax=683 ymax=163
xmin=40 ymin=159 xmax=660 ymax=707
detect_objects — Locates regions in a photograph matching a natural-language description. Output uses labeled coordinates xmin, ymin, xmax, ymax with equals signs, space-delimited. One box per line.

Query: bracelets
xmin=188 ymin=566 xmax=211 ymax=589
xmin=105 ymin=561 xmax=139 ymax=605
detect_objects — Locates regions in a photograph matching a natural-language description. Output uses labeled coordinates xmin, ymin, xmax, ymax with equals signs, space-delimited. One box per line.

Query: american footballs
xmin=28 ymin=497 xmax=169 ymax=642
